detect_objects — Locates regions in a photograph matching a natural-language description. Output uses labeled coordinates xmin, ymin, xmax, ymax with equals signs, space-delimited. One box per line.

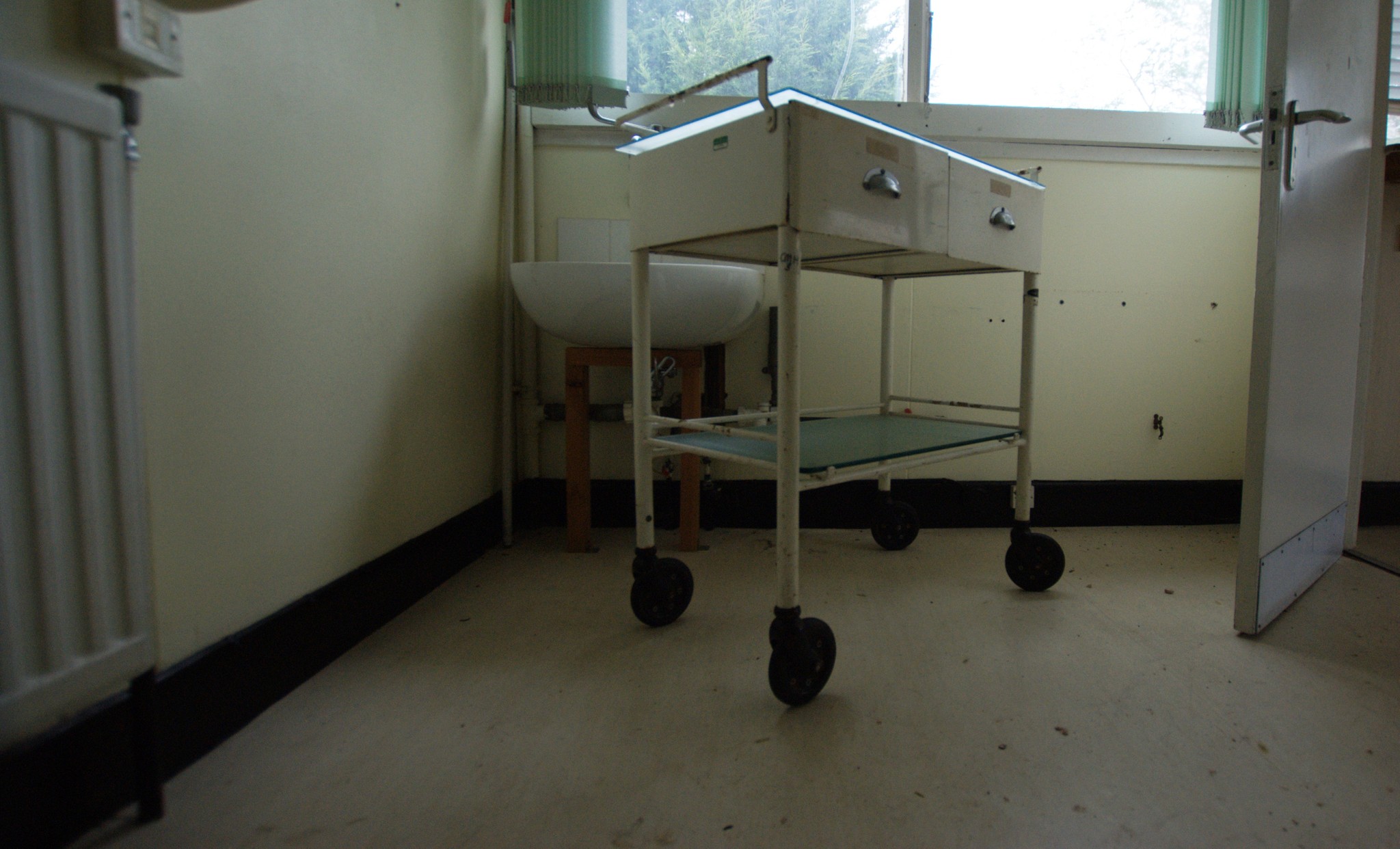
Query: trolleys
xmin=613 ymin=52 xmax=1068 ymax=709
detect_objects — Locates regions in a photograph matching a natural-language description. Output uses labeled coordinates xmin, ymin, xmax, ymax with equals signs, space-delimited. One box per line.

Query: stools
xmin=561 ymin=343 xmax=706 ymax=555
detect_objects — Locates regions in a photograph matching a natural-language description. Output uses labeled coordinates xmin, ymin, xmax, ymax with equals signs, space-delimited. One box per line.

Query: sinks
xmin=513 ymin=259 xmax=764 ymax=345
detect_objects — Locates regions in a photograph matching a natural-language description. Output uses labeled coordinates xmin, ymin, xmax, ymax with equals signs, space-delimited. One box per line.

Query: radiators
xmin=0 ymin=61 xmax=158 ymax=739
